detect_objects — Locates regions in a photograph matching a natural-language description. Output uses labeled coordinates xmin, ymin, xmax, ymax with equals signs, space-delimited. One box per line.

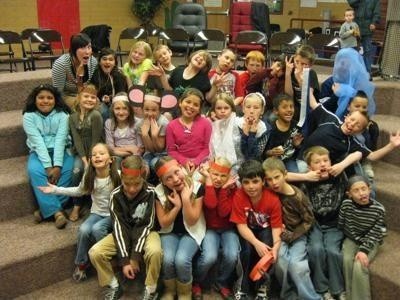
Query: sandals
xmin=34 ymin=210 xmax=44 ymax=222
xmin=68 ymin=206 xmax=81 ymax=222
xmin=54 ymin=211 xmax=67 ymax=229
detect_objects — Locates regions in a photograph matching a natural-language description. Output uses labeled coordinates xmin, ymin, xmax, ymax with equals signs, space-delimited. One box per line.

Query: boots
xmin=176 ymin=275 xmax=193 ymax=300
xmin=159 ymin=278 xmax=177 ymax=300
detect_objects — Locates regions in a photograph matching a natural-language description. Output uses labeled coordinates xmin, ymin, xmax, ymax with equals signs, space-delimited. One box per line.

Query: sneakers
xmin=319 ymin=292 xmax=348 ymax=300
xmin=72 ymin=264 xmax=87 ymax=283
xmin=191 ymin=282 xmax=203 ymax=300
xmin=211 ymin=280 xmax=235 ymax=300
xmin=234 ymin=285 xmax=271 ymax=300
xmin=104 ymin=279 xmax=124 ymax=300
xmin=142 ymin=285 xmax=159 ymax=300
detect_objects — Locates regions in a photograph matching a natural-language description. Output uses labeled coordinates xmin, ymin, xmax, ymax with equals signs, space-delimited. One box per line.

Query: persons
xmin=193 ymin=155 xmax=241 ymax=300
xmin=21 ymin=32 xmax=400 ymax=238
xmin=87 ymin=155 xmax=166 ymax=300
xmin=151 ymin=155 xmax=208 ymax=300
xmin=347 ymin=0 xmax=384 ymax=81
xmin=286 ymin=143 xmax=362 ymax=300
xmin=35 ymin=142 xmax=126 ymax=283
xmin=262 ymin=156 xmax=323 ymax=300
xmin=338 ymin=7 xmax=361 ymax=52
xmin=337 ymin=173 xmax=390 ymax=300
xmin=229 ymin=159 xmax=284 ymax=300
